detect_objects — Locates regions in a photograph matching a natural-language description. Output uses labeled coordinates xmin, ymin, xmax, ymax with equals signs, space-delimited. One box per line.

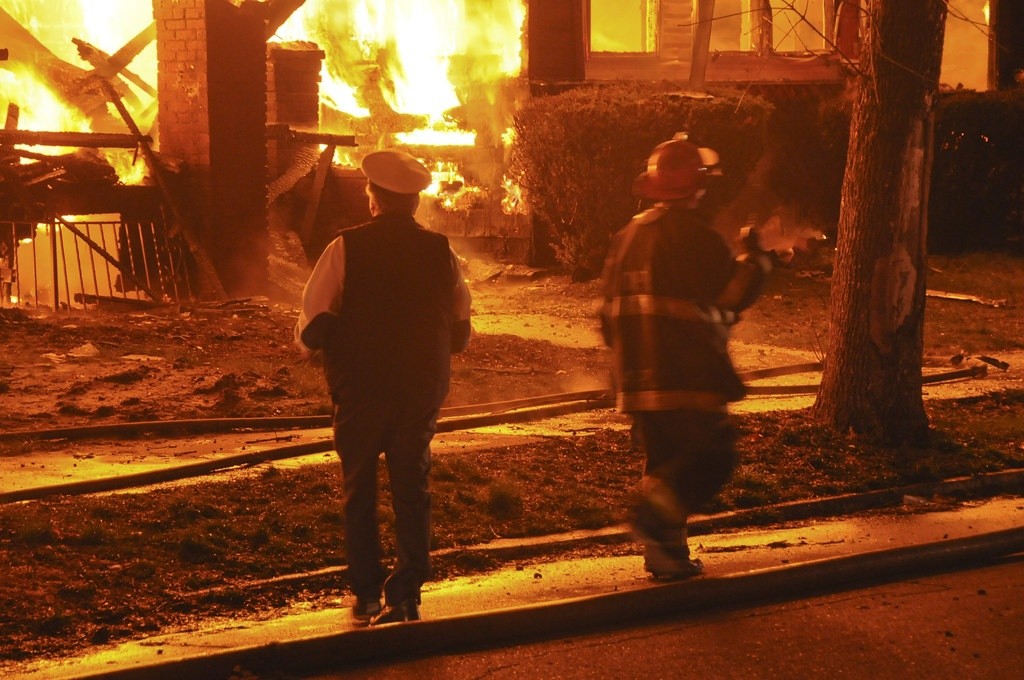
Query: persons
xmin=597 ymin=140 xmax=794 ymax=580
xmin=292 ymin=149 xmax=472 ymax=626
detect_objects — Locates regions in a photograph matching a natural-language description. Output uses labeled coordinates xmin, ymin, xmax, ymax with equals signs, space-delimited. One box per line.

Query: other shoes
xmin=352 ymin=595 xmax=382 ymax=620
xmin=643 ymin=550 xmax=702 ymax=578
xmin=623 ymin=499 xmax=689 ymax=559
xmin=368 ymin=596 xmax=420 ymax=626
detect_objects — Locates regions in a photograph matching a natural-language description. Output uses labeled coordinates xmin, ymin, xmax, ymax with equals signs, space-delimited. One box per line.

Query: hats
xmin=360 ymin=150 xmax=432 ymax=205
xmin=638 ymin=140 xmax=720 ymax=201
